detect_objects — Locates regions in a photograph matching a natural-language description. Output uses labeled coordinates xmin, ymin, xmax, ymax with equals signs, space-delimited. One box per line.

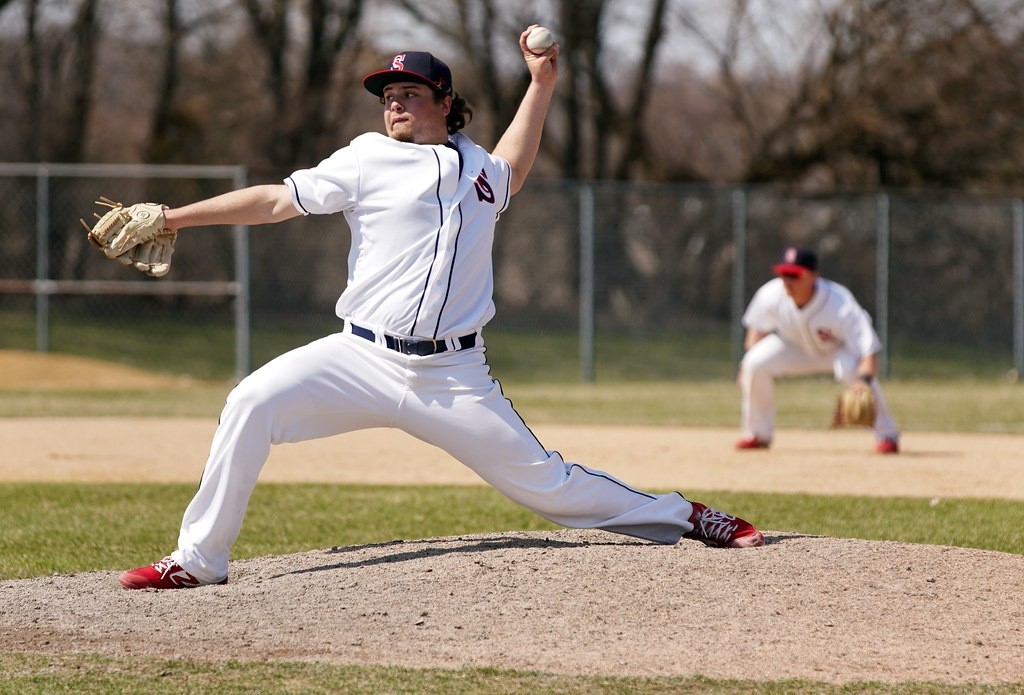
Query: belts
xmin=352 ymin=323 xmax=477 ymax=356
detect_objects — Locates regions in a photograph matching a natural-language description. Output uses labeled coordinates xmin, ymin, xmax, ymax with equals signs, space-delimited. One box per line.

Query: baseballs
xmin=525 ymin=26 xmax=555 ymax=56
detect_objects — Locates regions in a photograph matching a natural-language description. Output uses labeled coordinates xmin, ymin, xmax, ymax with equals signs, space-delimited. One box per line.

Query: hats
xmin=771 ymin=247 xmax=819 ymax=277
xmin=363 ymin=52 xmax=452 ymax=96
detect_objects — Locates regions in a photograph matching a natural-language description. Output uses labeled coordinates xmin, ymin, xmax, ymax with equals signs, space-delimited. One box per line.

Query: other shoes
xmin=736 ymin=438 xmax=768 ymax=448
xmin=876 ymin=438 xmax=898 ymax=455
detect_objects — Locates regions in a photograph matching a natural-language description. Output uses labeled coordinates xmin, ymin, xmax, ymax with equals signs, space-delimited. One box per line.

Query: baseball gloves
xmin=86 ymin=201 xmax=179 ymax=280
xmin=838 ymin=383 xmax=878 ymax=430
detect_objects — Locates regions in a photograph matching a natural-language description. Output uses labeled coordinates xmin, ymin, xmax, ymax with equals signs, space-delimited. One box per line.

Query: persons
xmin=736 ymin=247 xmax=901 ymax=454
xmin=80 ymin=24 xmax=765 ymax=591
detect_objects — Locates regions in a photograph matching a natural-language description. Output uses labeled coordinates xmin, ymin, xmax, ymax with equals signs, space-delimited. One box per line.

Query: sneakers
xmin=118 ymin=556 xmax=228 ymax=589
xmin=683 ymin=502 xmax=765 ymax=548
